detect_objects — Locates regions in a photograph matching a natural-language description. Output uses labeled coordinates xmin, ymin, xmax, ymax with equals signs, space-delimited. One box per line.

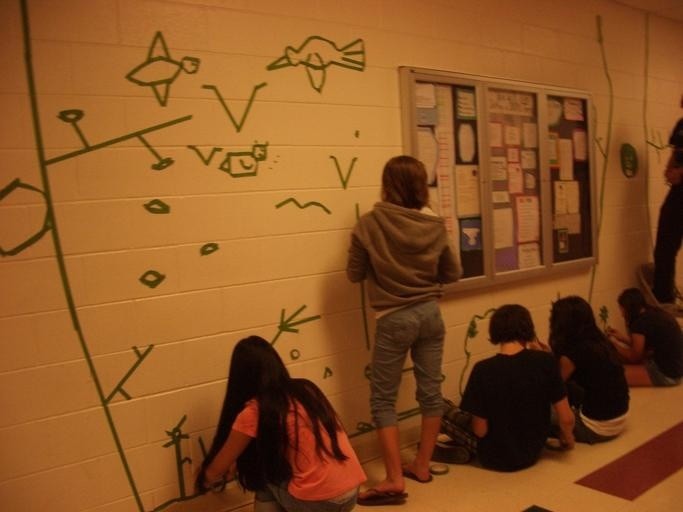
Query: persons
xmin=417 ymin=303 xmax=575 ymax=474
xmin=651 ymin=94 xmax=683 ymax=304
xmin=530 ymin=295 xmax=630 ymax=445
xmin=193 ymin=334 xmax=368 ymax=512
xmin=607 ymin=289 xmax=683 ymax=387
xmin=346 ymin=155 xmax=463 ymax=506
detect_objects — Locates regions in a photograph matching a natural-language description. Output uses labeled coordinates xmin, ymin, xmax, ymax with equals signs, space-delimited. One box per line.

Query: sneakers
xmin=416 ymin=440 xmax=470 ymax=465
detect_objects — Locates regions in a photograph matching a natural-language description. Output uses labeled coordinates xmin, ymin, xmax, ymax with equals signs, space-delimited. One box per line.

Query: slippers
xmin=356 ymin=488 xmax=408 ymax=504
xmin=399 ymin=461 xmax=432 ymax=483
